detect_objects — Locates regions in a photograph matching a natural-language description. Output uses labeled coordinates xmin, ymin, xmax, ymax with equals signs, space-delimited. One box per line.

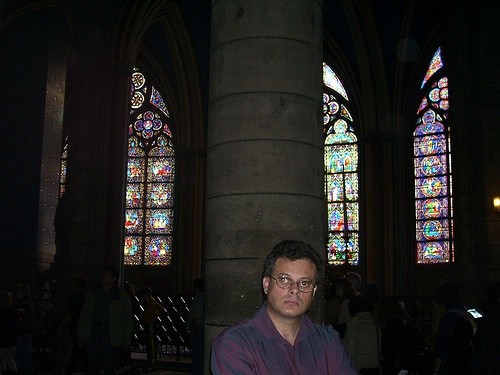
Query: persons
xmin=0 ymin=265 xmax=206 ymax=375
xmin=325 ymin=263 xmax=500 ymax=375
xmin=211 ymin=239 xmax=358 ymax=375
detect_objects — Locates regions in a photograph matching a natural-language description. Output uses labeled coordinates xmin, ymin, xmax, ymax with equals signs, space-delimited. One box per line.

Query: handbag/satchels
xmin=359 ymin=367 xmax=380 ymax=375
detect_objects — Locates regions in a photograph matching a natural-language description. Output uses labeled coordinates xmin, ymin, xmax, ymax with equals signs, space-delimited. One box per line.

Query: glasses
xmin=270 ymin=275 xmax=316 ymax=294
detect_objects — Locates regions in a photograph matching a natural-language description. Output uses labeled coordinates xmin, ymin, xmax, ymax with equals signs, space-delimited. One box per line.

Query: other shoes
xmin=148 ymin=368 xmax=153 ymax=371
